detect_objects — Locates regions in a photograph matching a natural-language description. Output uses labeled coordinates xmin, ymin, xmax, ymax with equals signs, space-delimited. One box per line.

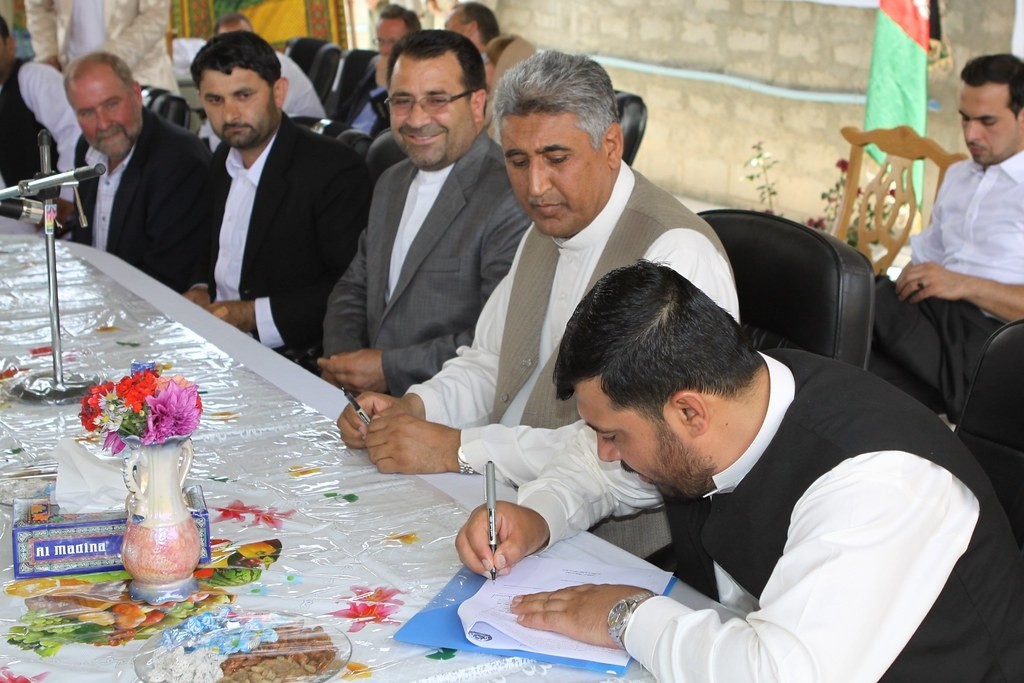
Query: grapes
xmin=15 ymin=610 xmax=81 ymax=634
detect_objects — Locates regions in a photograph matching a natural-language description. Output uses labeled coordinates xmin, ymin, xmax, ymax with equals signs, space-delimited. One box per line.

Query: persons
xmin=362 ymin=0 xmax=456 ymax=45
xmin=866 ymin=54 xmax=1024 ymax=413
xmin=456 ymin=262 xmax=1023 ymax=683
xmin=196 ymin=12 xmax=326 ymax=151
xmin=29 ymin=0 xmax=178 ymax=94
xmin=63 ymin=52 xmax=214 ymax=290
xmin=175 ymin=29 xmax=372 ymax=372
xmin=337 ymin=52 xmax=738 ymax=490
xmin=0 ymin=14 xmax=82 ymax=239
xmin=443 ymin=0 xmax=535 ymax=142
xmin=316 ymin=30 xmax=536 ymax=397
xmin=345 ymin=5 xmax=419 ymax=137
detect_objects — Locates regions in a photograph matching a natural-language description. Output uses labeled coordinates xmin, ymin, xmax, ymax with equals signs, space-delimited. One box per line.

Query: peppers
xmin=191 ymin=539 xmax=277 ymax=577
xmin=70 ymin=589 xmax=234 ymax=646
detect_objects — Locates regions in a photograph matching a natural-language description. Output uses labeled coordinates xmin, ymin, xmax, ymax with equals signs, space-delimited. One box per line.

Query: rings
xmin=918 ymin=279 xmax=923 ymax=287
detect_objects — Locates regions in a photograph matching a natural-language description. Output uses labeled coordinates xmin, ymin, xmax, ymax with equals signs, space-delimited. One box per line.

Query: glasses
xmin=384 ymin=87 xmax=477 ymax=114
xmin=373 ymin=38 xmax=399 ymax=48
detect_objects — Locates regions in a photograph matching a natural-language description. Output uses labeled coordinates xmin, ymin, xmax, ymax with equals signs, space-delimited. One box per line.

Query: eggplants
xmin=260 ymin=539 xmax=282 ymax=571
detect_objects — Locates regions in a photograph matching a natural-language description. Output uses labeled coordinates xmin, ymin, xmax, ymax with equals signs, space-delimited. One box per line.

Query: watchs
xmin=607 ymin=592 xmax=660 ymax=651
xmin=458 ymin=446 xmax=473 ymax=474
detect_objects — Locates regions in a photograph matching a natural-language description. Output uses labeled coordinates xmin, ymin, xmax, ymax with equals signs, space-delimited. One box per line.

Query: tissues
xmin=10 ymin=436 xmax=213 ymax=578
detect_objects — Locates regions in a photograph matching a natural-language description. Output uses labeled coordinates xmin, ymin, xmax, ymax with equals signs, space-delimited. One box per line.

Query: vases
xmin=122 ymin=434 xmax=204 ymax=605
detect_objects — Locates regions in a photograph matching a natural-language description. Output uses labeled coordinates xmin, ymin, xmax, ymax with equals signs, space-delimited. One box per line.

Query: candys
xmin=142 ymin=606 xmax=279 ymax=683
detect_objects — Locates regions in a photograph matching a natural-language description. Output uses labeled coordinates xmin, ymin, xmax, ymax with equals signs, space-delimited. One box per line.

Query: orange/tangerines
xmin=5 ymin=578 xmax=91 ymax=599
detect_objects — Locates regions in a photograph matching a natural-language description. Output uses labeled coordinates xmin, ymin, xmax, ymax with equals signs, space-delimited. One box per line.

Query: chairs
xmin=694 ymin=209 xmax=874 ymax=371
xmin=826 ymin=125 xmax=971 ymax=281
xmin=280 ymin=33 xmax=409 ymax=156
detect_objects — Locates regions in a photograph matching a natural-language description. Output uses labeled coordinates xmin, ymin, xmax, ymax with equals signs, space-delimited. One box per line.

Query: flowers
xmin=749 ymin=141 xmax=899 ymax=250
xmin=78 ymin=359 xmax=203 ymax=457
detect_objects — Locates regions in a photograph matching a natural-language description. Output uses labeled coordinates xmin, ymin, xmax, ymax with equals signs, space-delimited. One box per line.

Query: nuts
xmin=215 ymin=627 xmax=338 ymax=683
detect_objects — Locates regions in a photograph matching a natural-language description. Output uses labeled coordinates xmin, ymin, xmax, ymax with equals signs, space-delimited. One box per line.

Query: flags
xmin=863 ymin=0 xmax=930 ymax=235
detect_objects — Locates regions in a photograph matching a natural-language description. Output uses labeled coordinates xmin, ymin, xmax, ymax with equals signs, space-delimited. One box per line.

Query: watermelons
xmin=206 ymin=568 xmax=261 ymax=587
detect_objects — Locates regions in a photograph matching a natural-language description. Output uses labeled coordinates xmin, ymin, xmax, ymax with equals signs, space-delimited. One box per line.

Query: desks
xmin=0 ymin=233 xmax=751 ymax=683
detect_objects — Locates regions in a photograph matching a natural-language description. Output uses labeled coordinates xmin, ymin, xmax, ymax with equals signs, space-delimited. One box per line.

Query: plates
xmin=134 ymin=608 xmax=352 ymax=682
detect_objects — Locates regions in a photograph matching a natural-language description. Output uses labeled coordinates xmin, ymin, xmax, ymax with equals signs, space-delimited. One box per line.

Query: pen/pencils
xmin=484 ymin=461 xmax=496 ymax=584
xmin=342 ymin=387 xmax=370 ymax=425
